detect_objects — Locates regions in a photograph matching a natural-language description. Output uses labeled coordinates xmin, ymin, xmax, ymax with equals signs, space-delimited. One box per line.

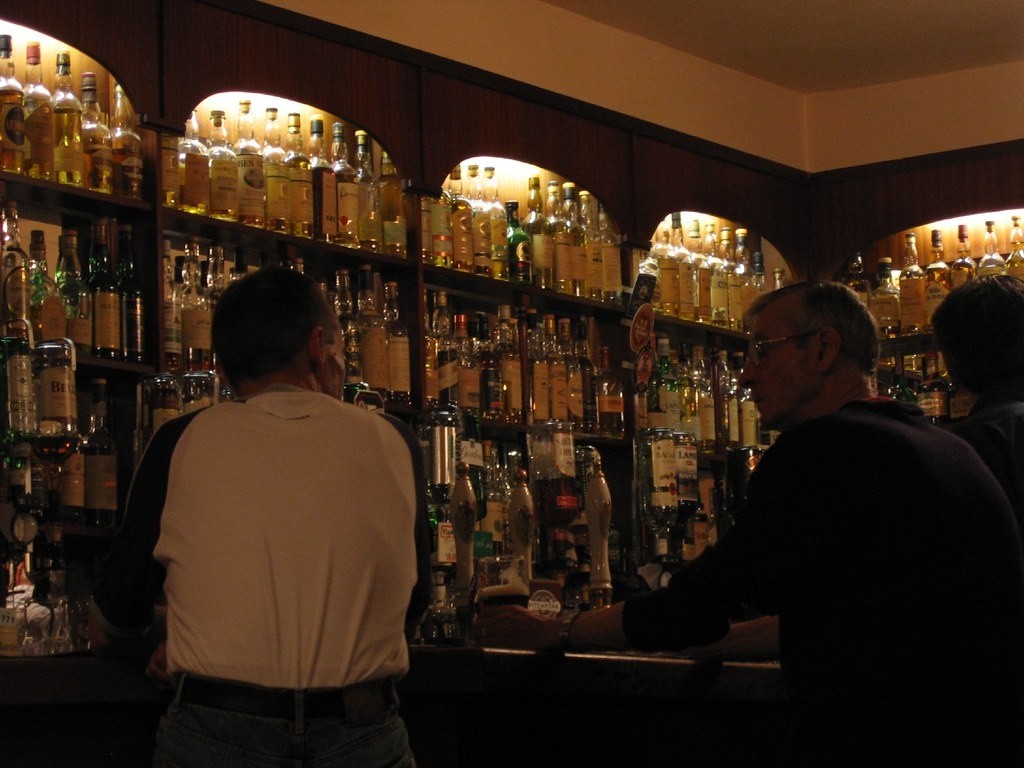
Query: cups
xmin=0 ymin=608 xmax=28 ymax=651
xmin=528 ymin=578 xmax=564 ymax=618
xmin=476 ymin=555 xmax=531 ymax=617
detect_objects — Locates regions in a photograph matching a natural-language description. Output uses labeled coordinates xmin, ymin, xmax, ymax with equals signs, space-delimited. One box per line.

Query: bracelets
xmin=558 ymin=610 xmax=580 ymax=653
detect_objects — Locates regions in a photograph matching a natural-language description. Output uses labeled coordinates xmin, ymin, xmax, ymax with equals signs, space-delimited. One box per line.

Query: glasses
xmin=742 ymin=331 xmax=816 ymax=367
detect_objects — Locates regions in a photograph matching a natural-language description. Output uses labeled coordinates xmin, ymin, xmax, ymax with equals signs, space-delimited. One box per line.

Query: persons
xmin=930 ymin=274 xmax=1024 ymax=539
xmin=83 ymin=266 xmax=433 ymax=768
xmin=471 ymin=284 xmax=1024 ymax=768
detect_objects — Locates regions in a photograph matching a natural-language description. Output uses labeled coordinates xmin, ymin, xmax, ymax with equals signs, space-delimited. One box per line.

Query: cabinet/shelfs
xmin=0 ymin=0 xmax=1024 ymax=654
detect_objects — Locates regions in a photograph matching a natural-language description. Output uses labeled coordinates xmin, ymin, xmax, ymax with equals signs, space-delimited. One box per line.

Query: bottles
xmin=420 ymin=164 xmax=623 ymax=304
xmin=0 ymin=339 xmax=122 ymax=530
xmin=160 ymin=236 xmax=412 ymax=404
xmin=0 ymin=197 xmax=151 ymax=366
xmin=141 ymin=370 xmax=243 ymax=451
xmin=424 ymin=348 xmax=626 ymax=568
xmin=158 ymin=100 xmax=407 ymax=260
xmin=422 ymin=288 xmax=626 ymax=436
xmin=888 ymin=350 xmax=977 ymax=425
xmin=636 ymin=426 xmax=764 ymax=563
xmin=635 ymin=211 xmax=786 ymax=335
xmin=635 ymin=338 xmax=780 ymax=447
xmin=845 ymin=215 xmax=1024 ymax=341
xmin=0 ymin=33 xmax=144 ymax=198
xmin=32 ymin=531 xmax=74 ymax=603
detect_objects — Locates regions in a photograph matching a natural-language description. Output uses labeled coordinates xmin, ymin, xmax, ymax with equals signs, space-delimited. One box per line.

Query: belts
xmin=176 ymin=675 xmax=397 ymax=720
